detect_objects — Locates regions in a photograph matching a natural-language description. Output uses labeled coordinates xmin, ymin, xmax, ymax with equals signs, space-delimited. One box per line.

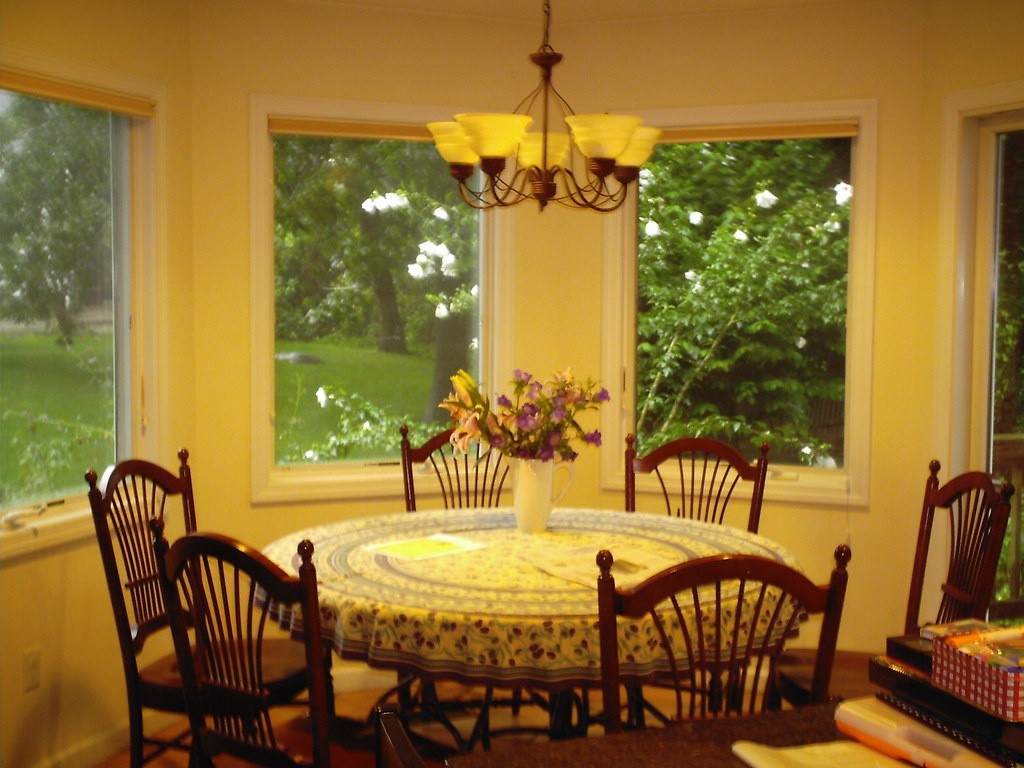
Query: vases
xmin=512 ymin=461 xmax=553 ymax=532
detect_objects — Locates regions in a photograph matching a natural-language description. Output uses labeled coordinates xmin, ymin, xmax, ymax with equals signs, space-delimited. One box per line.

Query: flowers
xmin=437 ymin=364 xmax=613 ymax=466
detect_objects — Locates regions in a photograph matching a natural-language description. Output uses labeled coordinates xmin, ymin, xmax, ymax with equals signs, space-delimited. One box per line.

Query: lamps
xmin=424 ymin=0 xmax=663 ymax=213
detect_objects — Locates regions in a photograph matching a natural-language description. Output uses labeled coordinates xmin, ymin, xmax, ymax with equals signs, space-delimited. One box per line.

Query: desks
xmin=235 ymin=506 xmax=811 ymax=741
xmin=372 ymin=691 xmax=1015 ymax=768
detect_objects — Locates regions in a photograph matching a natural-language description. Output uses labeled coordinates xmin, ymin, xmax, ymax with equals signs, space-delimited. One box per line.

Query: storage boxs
xmin=930 ymin=621 xmax=1024 ymax=721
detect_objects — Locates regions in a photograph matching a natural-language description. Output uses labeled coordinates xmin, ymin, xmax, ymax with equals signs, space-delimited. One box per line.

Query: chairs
xmin=904 ymin=458 xmax=1015 ymax=634
xmin=85 ymin=448 xmax=311 ymax=768
xmin=147 ymin=516 xmax=452 ymax=768
xmin=594 ymin=543 xmax=852 ymax=733
xmin=397 ymin=423 xmax=513 ymax=511
xmin=623 ymin=435 xmax=771 ymax=533
